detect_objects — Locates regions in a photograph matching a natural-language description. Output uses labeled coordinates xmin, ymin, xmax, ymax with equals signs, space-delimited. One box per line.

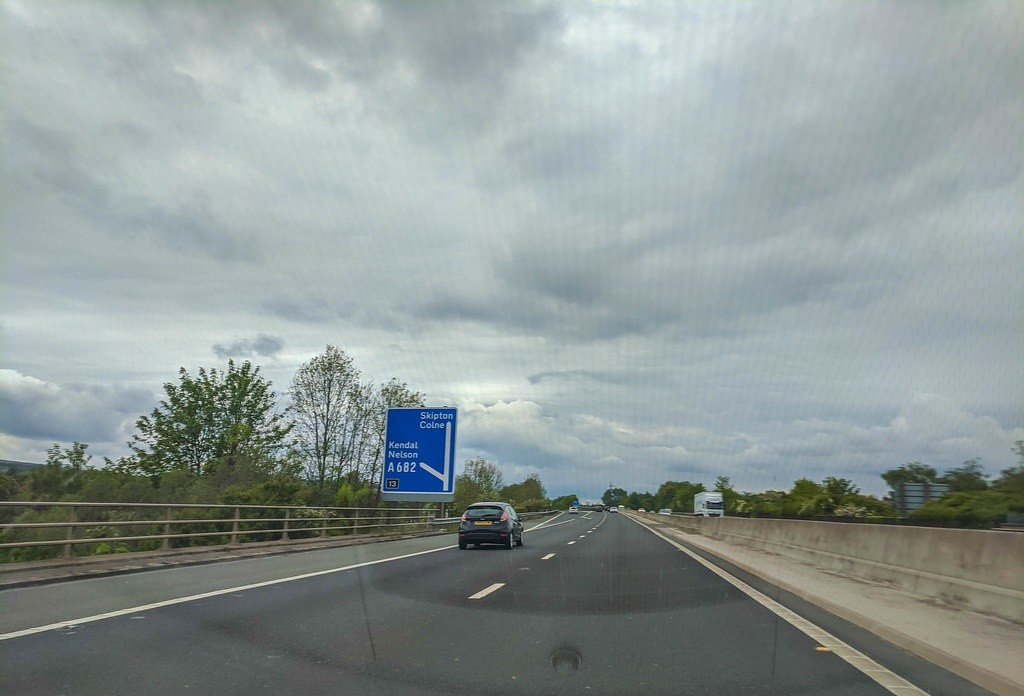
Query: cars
xmin=458 ymin=502 xmax=524 ymax=550
xmin=595 ymin=505 xmax=624 ymax=513
xmin=637 ymin=507 xmax=646 ymax=513
xmin=569 ymin=507 xmax=578 ymax=514
xmin=658 ymin=508 xmax=672 ymax=515
xmin=649 ymin=511 xmax=656 ymax=513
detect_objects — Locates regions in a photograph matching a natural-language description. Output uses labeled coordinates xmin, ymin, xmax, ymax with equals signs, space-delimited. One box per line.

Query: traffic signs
xmin=381 ymin=406 xmax=459 ymax=494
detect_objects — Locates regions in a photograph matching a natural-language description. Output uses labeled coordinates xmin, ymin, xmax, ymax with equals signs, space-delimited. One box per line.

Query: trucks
xmin=693 ymin=492 xmax=725 ymax=517
